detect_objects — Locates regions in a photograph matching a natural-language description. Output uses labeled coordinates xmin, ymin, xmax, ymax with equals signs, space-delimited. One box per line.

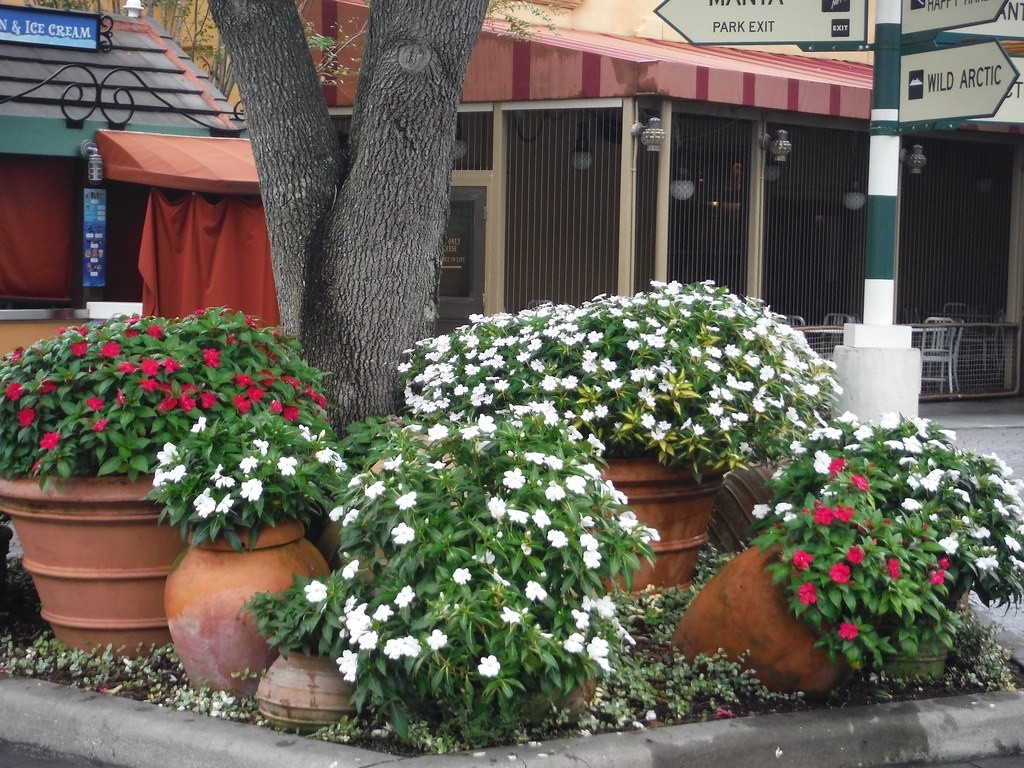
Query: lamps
xmin=631 ymin=116 xmax=665 ymax=152
xmin=454 ymin=124 xmax=468 ymax=161
xmin=842 ymin=167 xmax=867 ymax=210
xmin=761 ymin=129 xmax=792 ymax=162
xmin=570 ymin=122 xmax=594 ymax=171
xmin=670 ymin=159 xmax=694 ymax=200
xmin=899 ymin=144 xmax=927 ymax=174
xmin=79 ymin=138 xmax=104 ymax=185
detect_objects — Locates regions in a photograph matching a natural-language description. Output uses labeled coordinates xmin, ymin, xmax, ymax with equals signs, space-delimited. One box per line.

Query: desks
xmin=803 ymin=328 xmax=948 ymax=356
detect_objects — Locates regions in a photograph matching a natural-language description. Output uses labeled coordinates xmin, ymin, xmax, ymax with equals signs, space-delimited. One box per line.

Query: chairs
xmin=926 ymin=319 xmax=964 ymax=392
xmin=921 ymin=316 xmax=954 ymax=393
xmin=965 ymin=307 xmax=1004 ymax=386
xmin=820 ymin=313 xmax=853 ymax=361
xmin=943 ymin=303 xmax=970 ymax=323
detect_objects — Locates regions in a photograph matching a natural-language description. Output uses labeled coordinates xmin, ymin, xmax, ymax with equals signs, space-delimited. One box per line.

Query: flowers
xmin=145 ymin=410 xmax=354 ymax=575
xmin=749 ymin=410 xmax=1024 ymax=617
xmin=0 ymin=305 xmax=338 ymax=499
xmin=234 ymin=400 xmax=661 ymax=751
xmin=747 ymin=453 xmax=965 ymax=676
xmin=394 ymin=280 xmax=843 ymax=484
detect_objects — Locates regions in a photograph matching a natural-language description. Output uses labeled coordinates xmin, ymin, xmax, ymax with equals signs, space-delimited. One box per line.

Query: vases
xmin=872 ymin=572 xmax=970 ymax=680
xmin=0 ymin=476 xmax=189 ymax=659
xmin=165 ymin=518 xmax=330 ymax=700
xmin=673 ymin=545 xmax=846 ymax=694
xmin=597 ymin=460 xmax=731 ymax=596
xmin=253 ymin=647 xmax=357 ymax=733
